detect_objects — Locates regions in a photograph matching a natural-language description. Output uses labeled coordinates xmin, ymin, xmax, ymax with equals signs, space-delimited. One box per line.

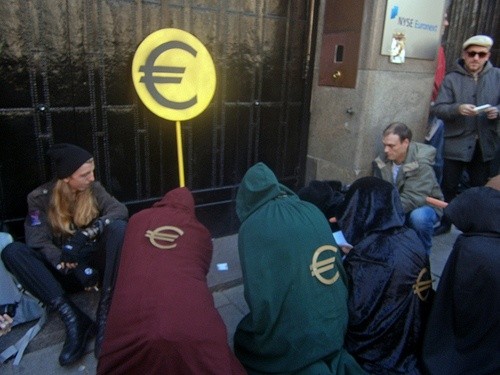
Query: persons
xmin=432 ymin=36 xmax=500 ymax=235
xmin=0 ymin=314 xmax=13 ymax=336
xmin=1 ymin=143 xmax=129 ymax=365
xmin=336 ymin=177 xmax=434 ymax=375
xmin=417 ymin=187 xmax=500 ymax=375
xmin=372 ymin=122 xmax=444 ymax=254
xmin=96 ymin=187 xmax=249 ymax=375
xmin=233 ymin=163 xmax=369 ymax=375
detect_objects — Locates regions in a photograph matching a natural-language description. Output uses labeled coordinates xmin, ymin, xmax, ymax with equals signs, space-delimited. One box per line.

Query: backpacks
xmin=297 ymin=179 xmax=350 ymax=231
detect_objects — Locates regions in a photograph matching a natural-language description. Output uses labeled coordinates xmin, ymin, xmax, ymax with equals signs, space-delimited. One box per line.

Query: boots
xmin=48 ymin=295 xmax=94 ymax=367
xmin=94 ymin=287 xmax=111 ymax=359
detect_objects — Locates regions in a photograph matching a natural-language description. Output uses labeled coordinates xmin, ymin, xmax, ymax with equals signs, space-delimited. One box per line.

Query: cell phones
xmin=474 ymin=104 xmax=491 ymax=114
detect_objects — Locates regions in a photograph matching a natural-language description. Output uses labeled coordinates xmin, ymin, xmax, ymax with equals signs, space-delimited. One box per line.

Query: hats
xmin=48 ymin=143 xmax=92 ymax=179
xmin=462 ymin=35 xmax=494 ymax=51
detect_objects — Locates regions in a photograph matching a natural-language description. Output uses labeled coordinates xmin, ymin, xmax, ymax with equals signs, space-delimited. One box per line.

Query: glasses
xmin=468 ymin=51 xmax=488 ymax=58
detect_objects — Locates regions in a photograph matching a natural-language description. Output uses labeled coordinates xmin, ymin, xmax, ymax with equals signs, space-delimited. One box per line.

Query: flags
xmin=424 ymin=46 xmax=446 ymax=185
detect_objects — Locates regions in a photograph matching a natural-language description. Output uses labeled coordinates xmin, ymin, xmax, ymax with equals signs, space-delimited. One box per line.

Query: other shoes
xmin=431 ymin=217 xmax=452 ymax=235
